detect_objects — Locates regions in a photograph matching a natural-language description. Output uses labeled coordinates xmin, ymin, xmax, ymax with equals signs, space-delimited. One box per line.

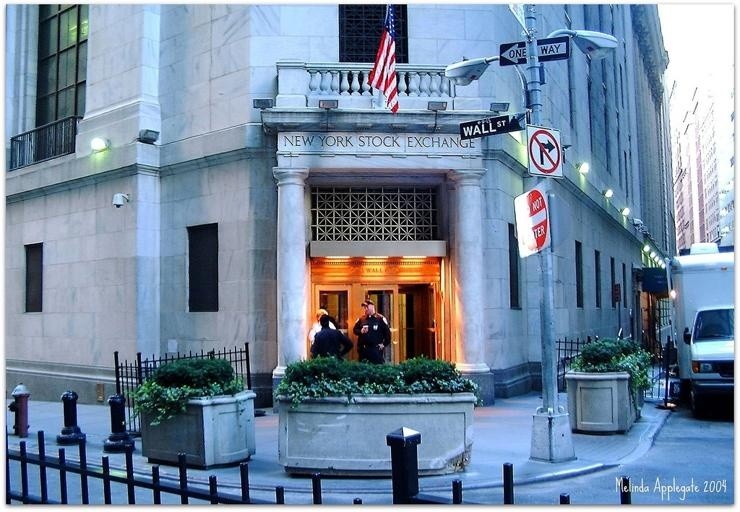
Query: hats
xmin=360 ymin=299 xmax=375 ymax=307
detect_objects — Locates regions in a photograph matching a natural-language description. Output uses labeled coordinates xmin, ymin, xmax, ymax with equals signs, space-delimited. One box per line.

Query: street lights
xmin=437 ymin=27 xmax=622 ymax=467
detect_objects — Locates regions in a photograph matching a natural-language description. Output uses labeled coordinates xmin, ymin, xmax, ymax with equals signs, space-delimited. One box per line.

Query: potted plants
xmin=275 ymin=354 xmax=478 ymax=481
xmin=132 ymin=353 xmax=260 ymax=469
xmin=563 ymin=334 xmax=655 ymax=433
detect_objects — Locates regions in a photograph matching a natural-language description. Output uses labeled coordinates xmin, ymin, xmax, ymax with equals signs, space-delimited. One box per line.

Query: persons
xmin=310 ymin=317 xmax=352 ymax=360
xmin=309 ymin=308 xmax=337 ymax=344
xmin=353 ymin=299 xmax=391 ymax=364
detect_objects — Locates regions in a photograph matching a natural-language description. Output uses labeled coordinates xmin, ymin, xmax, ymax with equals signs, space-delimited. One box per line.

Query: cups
xmin=363 ymin=326 xmax=368 ymax=334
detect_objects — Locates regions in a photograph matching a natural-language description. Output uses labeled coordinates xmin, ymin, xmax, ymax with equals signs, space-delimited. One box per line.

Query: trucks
xmin=660 ymin=241 xmax=735 ymax=423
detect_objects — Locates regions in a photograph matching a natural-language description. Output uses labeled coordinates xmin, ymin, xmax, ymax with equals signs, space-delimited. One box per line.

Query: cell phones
xmin=364 ymin=326 xmax=368 ymax=330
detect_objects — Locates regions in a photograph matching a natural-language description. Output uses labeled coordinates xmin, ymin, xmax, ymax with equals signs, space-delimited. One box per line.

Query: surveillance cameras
xmin=112 ymin=194 xmax=124 ymax=208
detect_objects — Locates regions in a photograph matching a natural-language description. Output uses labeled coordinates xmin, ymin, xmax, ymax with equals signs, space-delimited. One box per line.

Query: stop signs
xmin=514 ymin=183 xmax=551 ymax=260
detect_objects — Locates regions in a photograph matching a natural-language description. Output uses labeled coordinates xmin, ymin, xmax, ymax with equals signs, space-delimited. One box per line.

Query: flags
xmin=369 ymin=5 xmax=400 ymax=115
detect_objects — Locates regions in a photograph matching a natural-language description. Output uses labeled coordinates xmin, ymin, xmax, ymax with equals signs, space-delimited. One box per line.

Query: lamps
xmin=110 ymin=192 xmax=130 ymax=208
xmin=488 ymin=101 xmax=511 ymax=112
xmin=136 ymin=128 xmax=162 ymax=145
xmin=90 ymin=133 xmax=113 ymax=151
xmin=426 ymin=100 xmax=449 ymax=112
xmin=575 ymin=159 xmax=632 ymax=219
xmin=319 ymin=98 xmax=340 ymax=111
xmin=251 ymin=97 xmax=275 ymax=111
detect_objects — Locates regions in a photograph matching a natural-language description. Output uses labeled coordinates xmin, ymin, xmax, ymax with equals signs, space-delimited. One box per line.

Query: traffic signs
xmin=456 ymin=109 xmax=533 ymax=140
xmin=492 ymin=38 xmax=573 ymax=66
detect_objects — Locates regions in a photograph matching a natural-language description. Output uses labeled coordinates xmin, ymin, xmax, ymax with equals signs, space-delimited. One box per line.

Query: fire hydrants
xmin=9 ymin=383 xmax=38 ymax=440
